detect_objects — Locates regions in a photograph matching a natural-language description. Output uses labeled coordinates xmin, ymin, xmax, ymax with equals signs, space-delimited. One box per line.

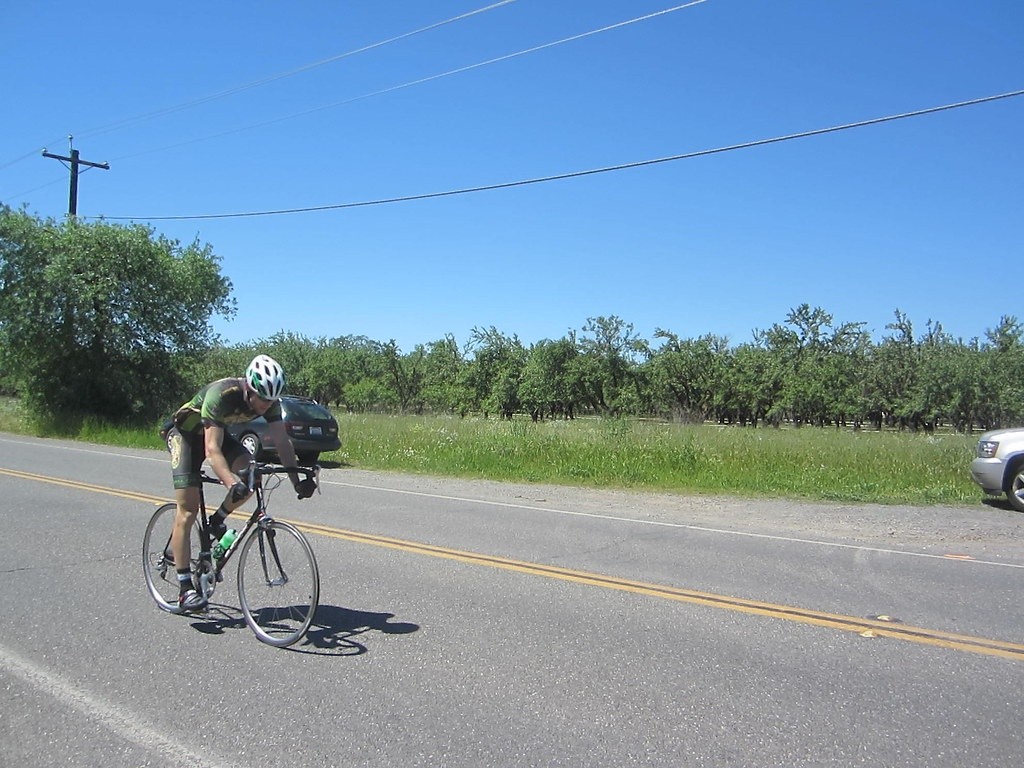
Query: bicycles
xmin=140 ymin=459 xmax=323 ymax=648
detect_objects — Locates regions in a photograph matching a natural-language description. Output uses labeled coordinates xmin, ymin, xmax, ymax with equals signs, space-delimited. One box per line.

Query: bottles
xmin=213 ymin=529 xmax=238 ymax=560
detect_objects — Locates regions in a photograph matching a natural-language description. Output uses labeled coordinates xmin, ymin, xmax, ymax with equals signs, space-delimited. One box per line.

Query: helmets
xmin=245 ymin=355 xmax=285 ymax=400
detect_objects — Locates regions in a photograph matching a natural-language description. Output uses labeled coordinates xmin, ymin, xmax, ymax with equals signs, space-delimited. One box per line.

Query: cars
xmin=158 ymin=394 xmax=341 ymax=464
xmin=969 ymin=425 xmax=1024 ymax=513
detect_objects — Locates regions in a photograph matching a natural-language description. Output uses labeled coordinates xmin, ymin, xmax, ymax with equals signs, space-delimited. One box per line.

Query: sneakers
xmin=207 ymin=515 xmax=226 ymax=542
xmin=179 ymin=586 xmax=208 ymax=611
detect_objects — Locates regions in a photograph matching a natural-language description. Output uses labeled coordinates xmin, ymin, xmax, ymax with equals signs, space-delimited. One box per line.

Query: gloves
xmin=295 ymin=480 xmax=316 ymax=497
xmin=230 ymin=483 xmax=249 ymax=502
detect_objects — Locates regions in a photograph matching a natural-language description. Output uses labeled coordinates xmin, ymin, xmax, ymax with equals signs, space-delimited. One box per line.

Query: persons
xmin=171 ymin=354 xmax=316 ymax=612
